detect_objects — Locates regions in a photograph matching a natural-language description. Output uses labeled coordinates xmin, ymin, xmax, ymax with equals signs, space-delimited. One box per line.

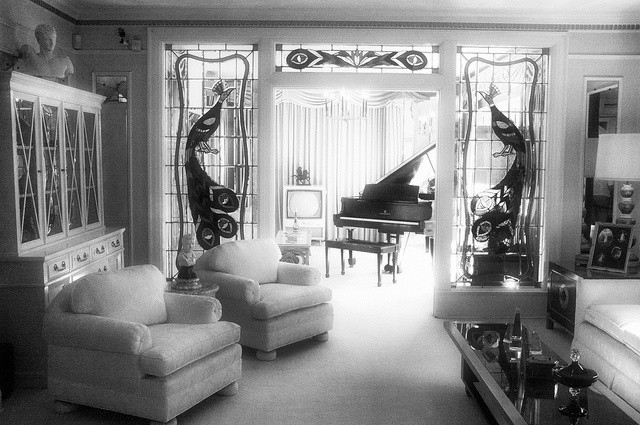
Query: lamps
xmin=595 ymin=132 xmax=640 ymax=266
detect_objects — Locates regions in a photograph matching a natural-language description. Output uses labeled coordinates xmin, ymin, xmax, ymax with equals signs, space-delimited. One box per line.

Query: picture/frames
xmin=587 ymin=221 xmax=634 ymax=273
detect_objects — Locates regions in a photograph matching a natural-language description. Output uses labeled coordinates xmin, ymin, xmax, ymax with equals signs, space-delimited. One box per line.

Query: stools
xmin=275 ymin=229 xmax=312 ymax=266
xmin=324 ymin=236 xmax=400 ymax=287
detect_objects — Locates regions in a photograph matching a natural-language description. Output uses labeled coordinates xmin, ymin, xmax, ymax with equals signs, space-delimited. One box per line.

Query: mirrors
xmin=91 ymin=71 xmax=133 ymax=267
xmin=581 ymin=85 xmax=620 ymax=256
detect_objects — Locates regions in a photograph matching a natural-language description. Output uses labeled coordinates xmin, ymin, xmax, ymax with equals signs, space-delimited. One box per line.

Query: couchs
xmin=194 ymin=238 xmax=334 ymax=362
xmin=570 ymin=278 xmax=640 ymax=424
xmin=41 ymin=264 xmax=243 ymax=425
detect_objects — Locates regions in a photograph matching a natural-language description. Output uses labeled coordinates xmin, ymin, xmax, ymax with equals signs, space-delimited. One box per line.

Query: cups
xmin=482 ymin=330 xmax=501 ymax=348
xmin=509 ymin=336 xmax=523 ymax=362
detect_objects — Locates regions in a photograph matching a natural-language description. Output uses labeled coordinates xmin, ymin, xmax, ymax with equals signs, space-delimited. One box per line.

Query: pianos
xmin=333 ymin=141 xmax=436 ymax=274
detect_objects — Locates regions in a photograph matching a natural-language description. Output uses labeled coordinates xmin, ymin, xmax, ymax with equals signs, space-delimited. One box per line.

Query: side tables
xmin=165 ymin=280 xmax=221 ymax=295
xmin=546 ymin=260 xmax=635 ymax=339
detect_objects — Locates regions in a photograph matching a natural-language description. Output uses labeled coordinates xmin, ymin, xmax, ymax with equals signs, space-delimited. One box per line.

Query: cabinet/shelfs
xmin=1 ymin=69 xmax=127 ymax=391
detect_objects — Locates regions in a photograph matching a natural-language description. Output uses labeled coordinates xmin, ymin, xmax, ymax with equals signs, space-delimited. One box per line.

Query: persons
xmin=20 ymin=24 xmax=74 ymax=79
xmin=177 ymin=233 xmax=196 ymax=267
